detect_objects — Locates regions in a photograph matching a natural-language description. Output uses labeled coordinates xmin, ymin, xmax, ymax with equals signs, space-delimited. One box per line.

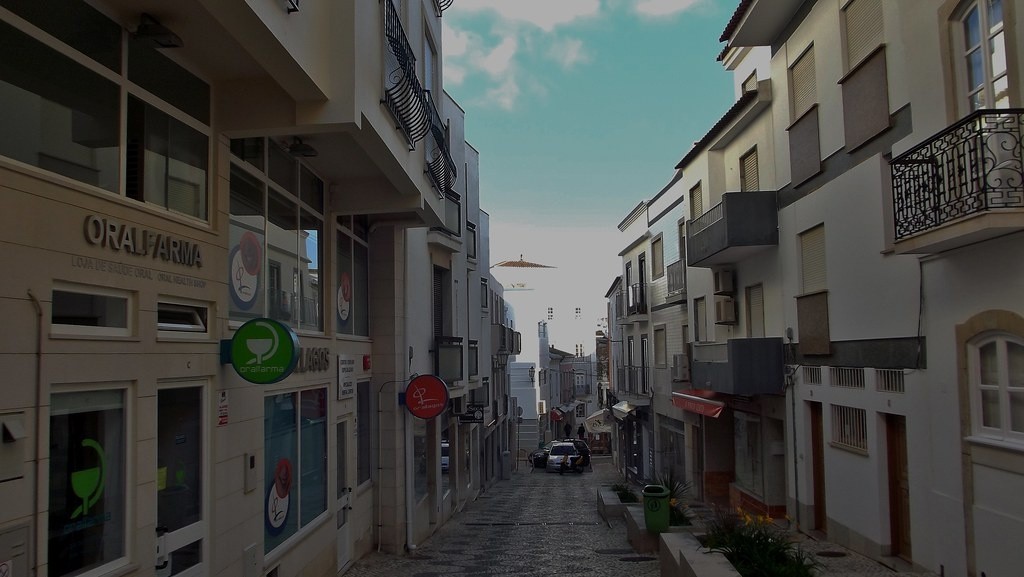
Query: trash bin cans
xmin=641 ymin=484 xmax=671 ymax=536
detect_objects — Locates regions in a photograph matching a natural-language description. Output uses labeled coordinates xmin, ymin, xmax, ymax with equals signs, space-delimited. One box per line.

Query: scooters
xmin=556 ymin=451 xmax=585 ymax=473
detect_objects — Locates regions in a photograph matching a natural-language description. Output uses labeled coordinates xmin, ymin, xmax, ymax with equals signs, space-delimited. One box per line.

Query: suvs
xmin=528 ymin=438 xmax=591 ymax=467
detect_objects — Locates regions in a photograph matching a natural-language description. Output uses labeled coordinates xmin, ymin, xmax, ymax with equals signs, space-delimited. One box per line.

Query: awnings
xmin=551 ymin=399 xmax=581 ymax=421
xmin=612 ymin=401 xmax=638 ymax=422
xmin=671 ymin=390 xmax=738 ymax=419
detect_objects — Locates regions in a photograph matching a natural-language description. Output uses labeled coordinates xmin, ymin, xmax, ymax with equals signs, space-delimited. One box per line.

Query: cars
xmin=548 ymin=441 xmax=582 ymax=472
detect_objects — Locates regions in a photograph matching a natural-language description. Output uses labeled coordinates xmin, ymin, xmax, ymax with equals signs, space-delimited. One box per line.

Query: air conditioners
xmin=672 ymin=353 xmax=689 ymax=381
xmin=713 ymin=267 xmax=733 ymax=293
xmin=714 ymin=298 xmax=735 ymax=322
xmin=538 ymin=399 xmax=547 ymax=415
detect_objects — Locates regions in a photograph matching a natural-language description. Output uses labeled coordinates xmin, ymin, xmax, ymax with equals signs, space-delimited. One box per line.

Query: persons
xmin=564 ymin=421 xmax=572 ymax=437
xmin=577 ymin=422 xmax=585 ymax=440
